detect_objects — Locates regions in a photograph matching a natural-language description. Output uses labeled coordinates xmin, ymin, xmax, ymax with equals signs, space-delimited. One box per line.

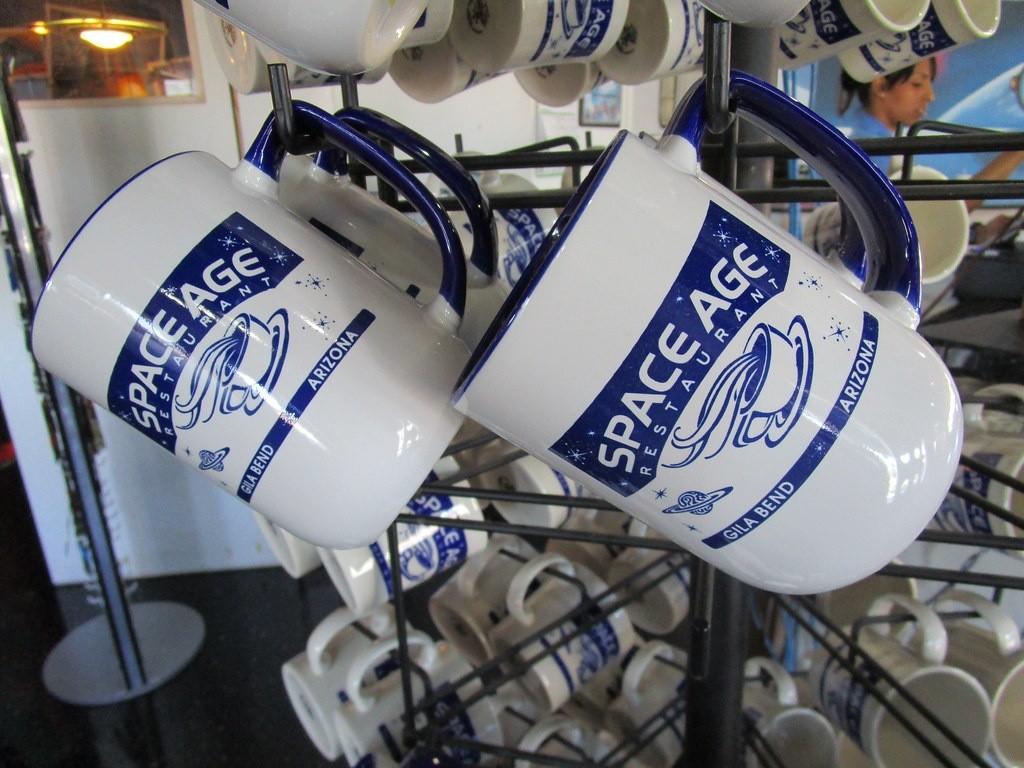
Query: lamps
xmin=45 ymin=0 xmax=168 ymax=50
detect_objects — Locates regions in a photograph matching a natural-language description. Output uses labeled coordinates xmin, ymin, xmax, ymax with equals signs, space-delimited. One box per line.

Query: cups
xmin=250 ymin=381 xmax=1024 ymax=768
xmin=799 ymin=164 xmax=969 ymax=291
xmin=26 ymin=99 xmax=516 ymax=552
xmin=196 ymin=0 xmax=1002 ymax=110
xmin=441 ymin=66 xmax=960 ymax=597
xmin=436 ymin=152 xmax=559 ymax=283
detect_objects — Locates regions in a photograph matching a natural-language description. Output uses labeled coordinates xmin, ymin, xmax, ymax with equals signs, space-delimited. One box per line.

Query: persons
xmin=812 ymin=52 xmax=1024 ymax=244
